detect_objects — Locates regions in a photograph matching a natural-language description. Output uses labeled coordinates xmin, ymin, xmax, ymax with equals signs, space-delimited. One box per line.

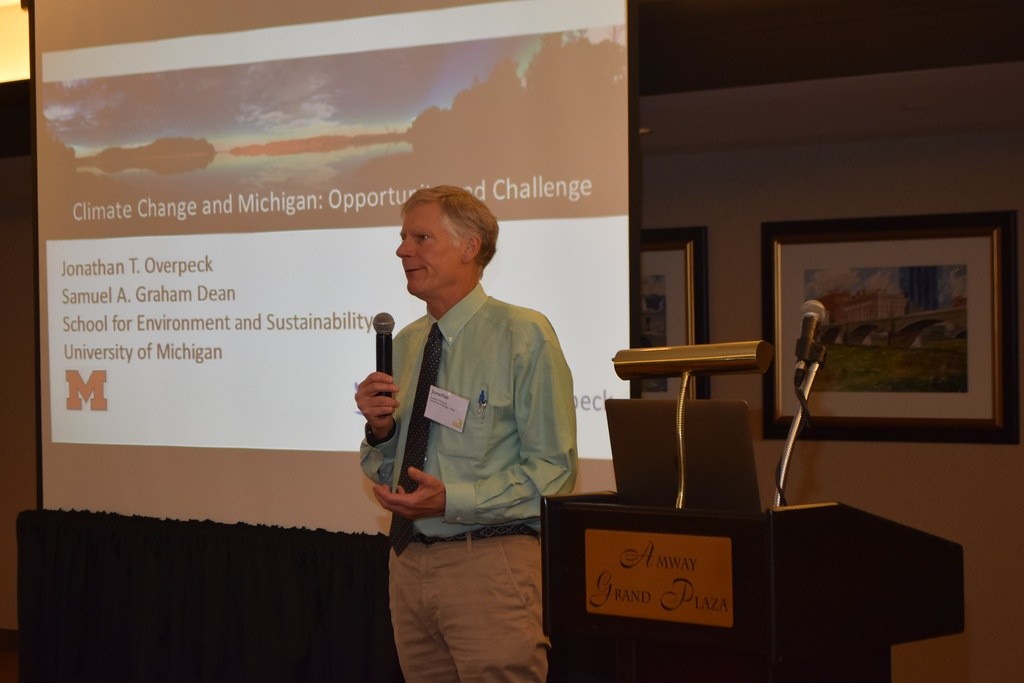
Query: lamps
xmin=613 ymin=339 xmax=773 ymax=508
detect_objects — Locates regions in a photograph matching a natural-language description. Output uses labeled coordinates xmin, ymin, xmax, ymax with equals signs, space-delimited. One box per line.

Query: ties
xmin=388 ymin=321 xmax=443 ymax=557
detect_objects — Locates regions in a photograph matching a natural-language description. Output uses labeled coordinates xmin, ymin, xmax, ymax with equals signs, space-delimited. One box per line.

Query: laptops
xmin=606 ymin=398 xmax=762 ymax=512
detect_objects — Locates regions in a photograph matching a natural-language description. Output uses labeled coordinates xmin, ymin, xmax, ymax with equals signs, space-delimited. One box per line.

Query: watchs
xmin=365 ymin=417 xmax=397 ymax=447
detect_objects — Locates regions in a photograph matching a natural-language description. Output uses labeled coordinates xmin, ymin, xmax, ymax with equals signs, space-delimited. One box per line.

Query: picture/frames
xmin=640 ymin=224 xmax=710 ymax=399
xmin=761 ymin=209 xmax=1020 ymax=445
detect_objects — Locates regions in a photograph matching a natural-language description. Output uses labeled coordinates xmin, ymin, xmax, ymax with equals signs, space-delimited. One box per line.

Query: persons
xmin=353 ymin=185 xmax=578 ymax=683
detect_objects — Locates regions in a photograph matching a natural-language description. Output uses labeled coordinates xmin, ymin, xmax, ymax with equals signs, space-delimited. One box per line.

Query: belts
xmin=409 ymin=524 xmax=539 ymax=545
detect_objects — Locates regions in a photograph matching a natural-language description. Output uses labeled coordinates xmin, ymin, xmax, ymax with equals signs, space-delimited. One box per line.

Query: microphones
xmin=794 ymin=298 xmax=826 ymax=387
xmin=373 ymin=312 xmax=395 ymax=418
